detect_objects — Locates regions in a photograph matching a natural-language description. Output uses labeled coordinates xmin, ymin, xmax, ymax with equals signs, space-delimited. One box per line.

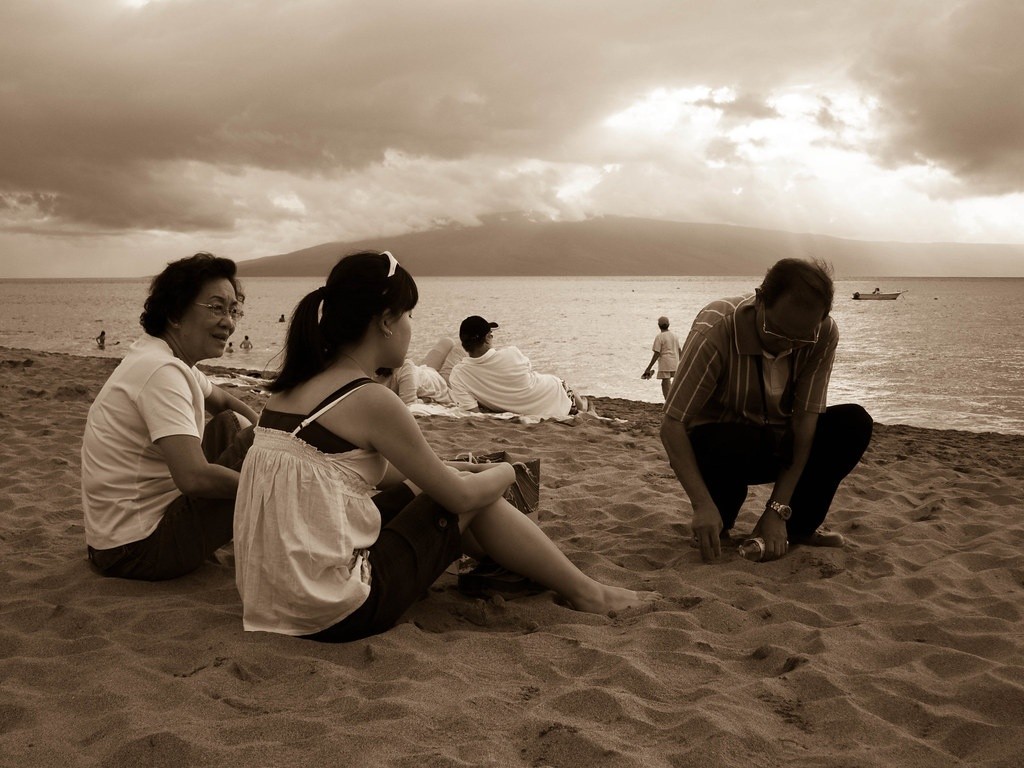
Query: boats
xmin=852 ymin=289 xmax=909 ymax=300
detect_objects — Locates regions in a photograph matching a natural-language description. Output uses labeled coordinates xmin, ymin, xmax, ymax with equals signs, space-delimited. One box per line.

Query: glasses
xmin=379 ymin=251 xmax=401 ymax=300
xmin=763 ymin=302 xmax=821 ymax=345
xmin=192 ymin=299 xmax=244 ymax=322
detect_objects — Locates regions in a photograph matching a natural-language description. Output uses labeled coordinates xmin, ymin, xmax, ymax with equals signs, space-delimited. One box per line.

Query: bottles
xmin=737 ymin=538 xmax=792 ymax=560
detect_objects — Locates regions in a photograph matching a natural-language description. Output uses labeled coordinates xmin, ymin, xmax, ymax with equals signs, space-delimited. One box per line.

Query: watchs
xmin=767 ymin=501 xmax=792 ymax=520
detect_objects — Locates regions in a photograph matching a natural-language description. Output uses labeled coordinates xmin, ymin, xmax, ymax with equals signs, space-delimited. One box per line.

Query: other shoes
xmin=458 ymin=555 xmax=539 ymax=599
xmin=690 ymin=531 xmax=734 ymax=547
xmin=641 ymin=370 xmax=654 ymax=380
xmin=802 ymin=530 xmax=845 ymax=547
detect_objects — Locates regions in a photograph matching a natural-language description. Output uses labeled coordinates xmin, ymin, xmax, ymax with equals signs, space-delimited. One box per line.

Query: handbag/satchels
xmin=455 ymin=451 xmax=541 ymax=527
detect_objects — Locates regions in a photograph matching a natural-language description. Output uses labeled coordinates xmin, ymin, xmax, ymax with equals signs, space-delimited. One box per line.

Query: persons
xmin=874 ymin=288 xmax=880 ymax=294
xmin=643 ymin=317 xmax=682 ymax=400
xmin=226 ymin=342 xmax=233 ymax=353
xmin=234 ymin=251 xmax=664 ymax=641
xmin=279 ymin=315 xmax=285 ymax=322
xmin=240 ymin=336 xmax=252 ymax=349
xmin=96 ymin=330 xmax=105 ymax=349
xmin=448 ymin=316 xmax=588 ymax=419
xmin=660 ymin=257 xmax=874 ymax=561
xmin=370 ymin=336 xmax=468 ymax=407
xmin=81 ymin=253 xmax=258 ymax=583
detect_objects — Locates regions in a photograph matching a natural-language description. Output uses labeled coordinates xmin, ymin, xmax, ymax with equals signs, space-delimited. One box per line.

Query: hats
xmin=658 ymin=316 xmax=670 ymax=327
xmin=460 ymin=316 xmax=498 ymax=344
